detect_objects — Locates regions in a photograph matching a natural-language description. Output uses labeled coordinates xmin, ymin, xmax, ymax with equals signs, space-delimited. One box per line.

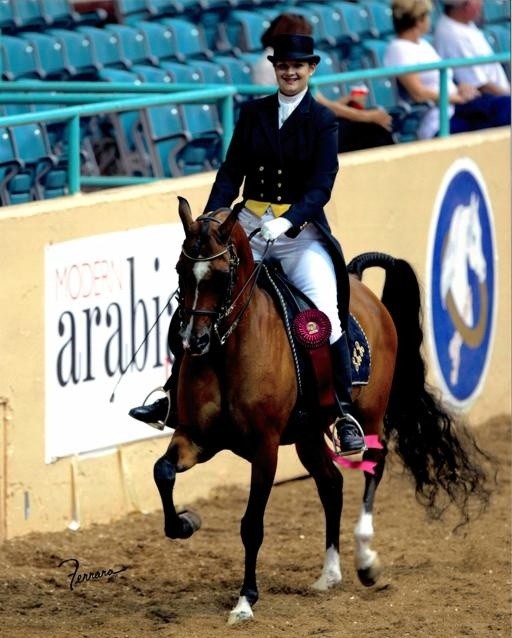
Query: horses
xmin=152 ymin=195 xmax=502 ymax=627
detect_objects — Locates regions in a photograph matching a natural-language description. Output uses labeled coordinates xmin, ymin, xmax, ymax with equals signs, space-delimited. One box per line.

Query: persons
xmin=384 ymin=0 xmax=511 ymax=136
xmin=254 ymin=13 xmax=393 ymax=153
xmin=130 ymin=61 xmax=365 ymax=452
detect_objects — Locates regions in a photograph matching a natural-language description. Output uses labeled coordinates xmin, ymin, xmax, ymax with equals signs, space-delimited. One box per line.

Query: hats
xmin=267 ymin=34 xmax=320 ymax=65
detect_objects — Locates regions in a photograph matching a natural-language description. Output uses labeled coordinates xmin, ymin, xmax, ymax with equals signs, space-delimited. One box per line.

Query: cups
xmin=350 ymin=86 xmax=369 ymax=110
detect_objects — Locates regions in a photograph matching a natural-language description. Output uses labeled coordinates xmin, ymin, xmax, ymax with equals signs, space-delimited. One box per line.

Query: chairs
xmin=1 ymin=0 xmax=511 ymax=205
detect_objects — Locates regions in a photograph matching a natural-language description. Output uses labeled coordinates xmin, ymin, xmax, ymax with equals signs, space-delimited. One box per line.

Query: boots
xmin=329 ymin=331 xmax=365 ymax=452
xmin=129 ymin=397 xmax=176 ymax=429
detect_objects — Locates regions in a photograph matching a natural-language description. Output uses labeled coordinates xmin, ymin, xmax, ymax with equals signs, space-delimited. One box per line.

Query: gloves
xmin=261 ymin=217 xmax=292 ymax=241
